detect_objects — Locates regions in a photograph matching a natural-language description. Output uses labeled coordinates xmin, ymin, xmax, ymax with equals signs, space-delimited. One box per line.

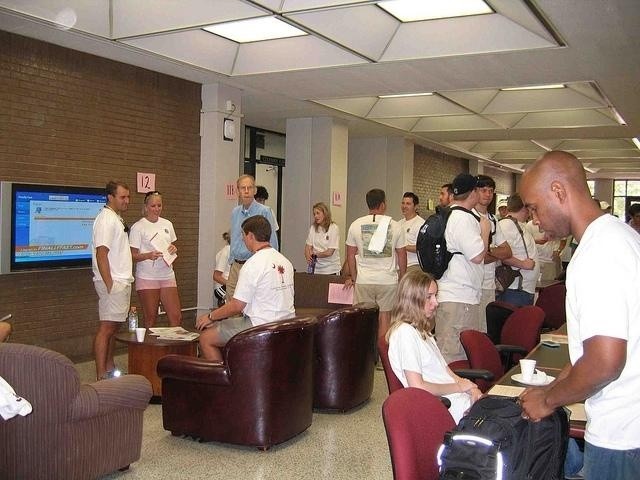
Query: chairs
xmin=0 ymin=340 xmax=150 ymax=479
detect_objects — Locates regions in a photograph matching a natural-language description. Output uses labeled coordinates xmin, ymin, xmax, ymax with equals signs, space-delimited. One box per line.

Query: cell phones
xmin=0 ymin=314 xmax=13 ymax=321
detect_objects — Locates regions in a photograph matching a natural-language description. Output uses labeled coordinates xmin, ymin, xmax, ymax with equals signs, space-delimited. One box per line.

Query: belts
xmin=234 ymin=258 xmax=245 ymax=264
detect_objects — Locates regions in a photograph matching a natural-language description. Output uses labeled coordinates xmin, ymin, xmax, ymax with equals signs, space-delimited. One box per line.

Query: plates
xmin=511 ymin=373 xmax=556 ymax=387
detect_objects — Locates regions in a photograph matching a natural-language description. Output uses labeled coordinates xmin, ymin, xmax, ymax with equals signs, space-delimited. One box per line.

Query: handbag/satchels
xmin=496 ymin=265 xmax=523 ymax=292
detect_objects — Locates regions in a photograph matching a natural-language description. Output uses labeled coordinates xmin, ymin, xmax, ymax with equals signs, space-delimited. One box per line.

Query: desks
xmin=478 ymin=321 xmax=585 ymax=439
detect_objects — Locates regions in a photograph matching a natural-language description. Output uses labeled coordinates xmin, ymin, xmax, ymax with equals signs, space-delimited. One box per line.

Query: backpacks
xmin=437 ymin=395 xmax=570 ymax=480
xmin=416 ymin=206 xmax=461 ymax=279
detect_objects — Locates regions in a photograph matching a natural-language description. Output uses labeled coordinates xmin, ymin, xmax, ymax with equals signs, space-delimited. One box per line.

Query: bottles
xmin=307 ymin=251 xmax=317 ymax=273
xmin=128 ymin=305 xmax=139 ymax=335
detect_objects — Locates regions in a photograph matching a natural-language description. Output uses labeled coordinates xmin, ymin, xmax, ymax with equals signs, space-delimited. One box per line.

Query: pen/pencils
xmin=153 ymin=261 xmax=154 ymax=267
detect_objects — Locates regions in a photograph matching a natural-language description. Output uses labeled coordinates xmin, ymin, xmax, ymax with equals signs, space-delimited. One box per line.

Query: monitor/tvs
xmin=1 ymin=180 xmax=121 ymax=276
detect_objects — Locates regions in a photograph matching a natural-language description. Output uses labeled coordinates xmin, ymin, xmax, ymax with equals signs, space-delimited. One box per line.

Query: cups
xmin=134 ymin=328 xmax=147 ymax=344
xmin=519 ymin=358 xmax=537 ymax=381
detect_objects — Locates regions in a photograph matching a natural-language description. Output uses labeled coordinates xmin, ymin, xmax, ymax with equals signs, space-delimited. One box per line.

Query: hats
xmin=452 ymin=174 xmax=473 ymax=194
xmin=600 ymin=201 xmax=612 ymax=210
xmin=497 ymin=199 xmax=508 ymax=209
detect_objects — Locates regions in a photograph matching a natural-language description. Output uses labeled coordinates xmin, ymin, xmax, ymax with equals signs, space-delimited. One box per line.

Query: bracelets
xmin=207 ymin=312 xmax=214 ymax=323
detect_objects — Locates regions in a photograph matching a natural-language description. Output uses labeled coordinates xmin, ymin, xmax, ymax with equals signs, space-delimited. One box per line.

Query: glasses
xmin=473 ymin=175 xmax=480 ymax=189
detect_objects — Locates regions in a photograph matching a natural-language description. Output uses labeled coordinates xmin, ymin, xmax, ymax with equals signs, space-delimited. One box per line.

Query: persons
xmin=89 ymin=180 xmax=136 ymax=381
xmin=224 ymin=174 xmax=280 ymax=321
xmin=514 ymin=148 xmax=639 ymax=479
xmin=305 ymin=174 xmax=567 ymax=365
xmin=387 ymin=269 xmax=484 ymax=426
xmin=213 ymin=230 xmax=234 ymax=305
xmin=193 ymin=215 xmax=298 ymax=364
xmin=594 ymin=198 xmax=640 ymax=231
xmin=128 ymin=189 xmax=183 ymax=329
xmin=253 ymin=185 xmax=268 ymax=206
